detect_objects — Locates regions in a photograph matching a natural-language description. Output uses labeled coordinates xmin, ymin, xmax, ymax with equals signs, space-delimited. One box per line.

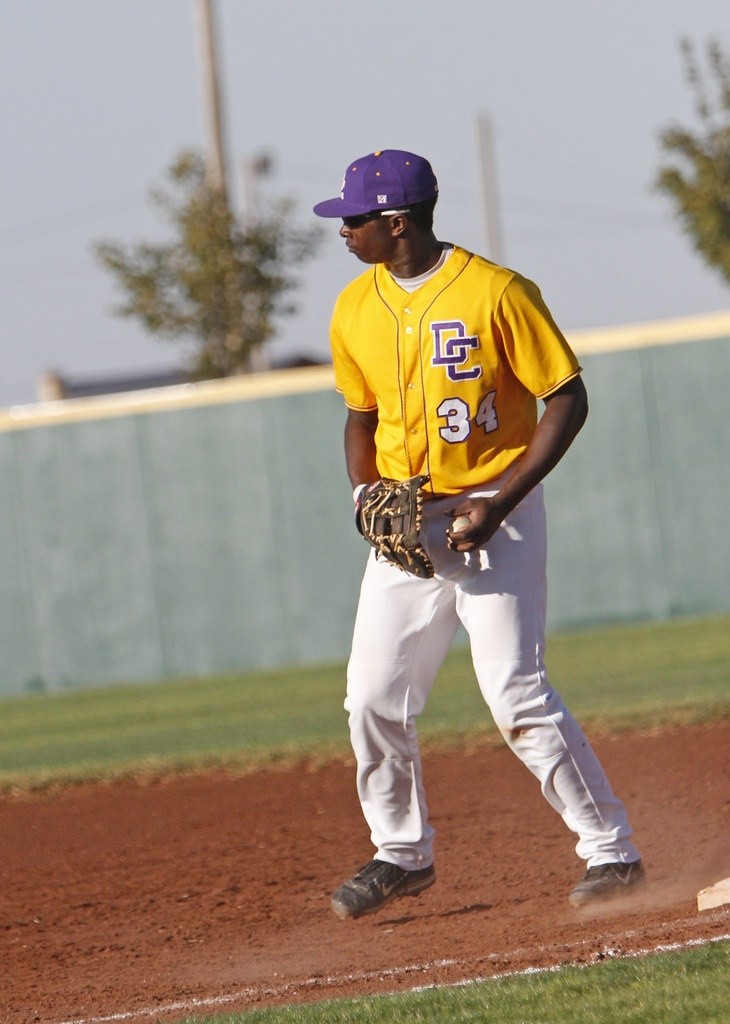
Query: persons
xmin=314 ymin=148 xmax=648 ymax=919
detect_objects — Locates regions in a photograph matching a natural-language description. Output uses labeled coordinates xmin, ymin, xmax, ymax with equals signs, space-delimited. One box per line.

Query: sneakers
xmin=331 ymin=859 xmax=437 ymax=915
xmin=568 ymin=858 xmax=646 ymax=907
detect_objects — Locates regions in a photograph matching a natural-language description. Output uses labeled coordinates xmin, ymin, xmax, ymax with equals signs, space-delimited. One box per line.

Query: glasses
xmin=341 ymin=209 xmax=410 ymax=227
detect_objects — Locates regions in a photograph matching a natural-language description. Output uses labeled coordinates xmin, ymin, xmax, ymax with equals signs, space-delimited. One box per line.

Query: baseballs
xmin=449 ymin=516 xmax=471 ymax=533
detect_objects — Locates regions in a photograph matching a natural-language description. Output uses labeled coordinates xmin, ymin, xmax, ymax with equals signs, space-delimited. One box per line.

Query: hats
xmin=313 ymin=150 xmax=439 ymax=218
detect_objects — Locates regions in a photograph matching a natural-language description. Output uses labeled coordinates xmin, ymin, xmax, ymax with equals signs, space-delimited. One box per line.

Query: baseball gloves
xmin=354 ymin=474 xmax=435 ymax=579
